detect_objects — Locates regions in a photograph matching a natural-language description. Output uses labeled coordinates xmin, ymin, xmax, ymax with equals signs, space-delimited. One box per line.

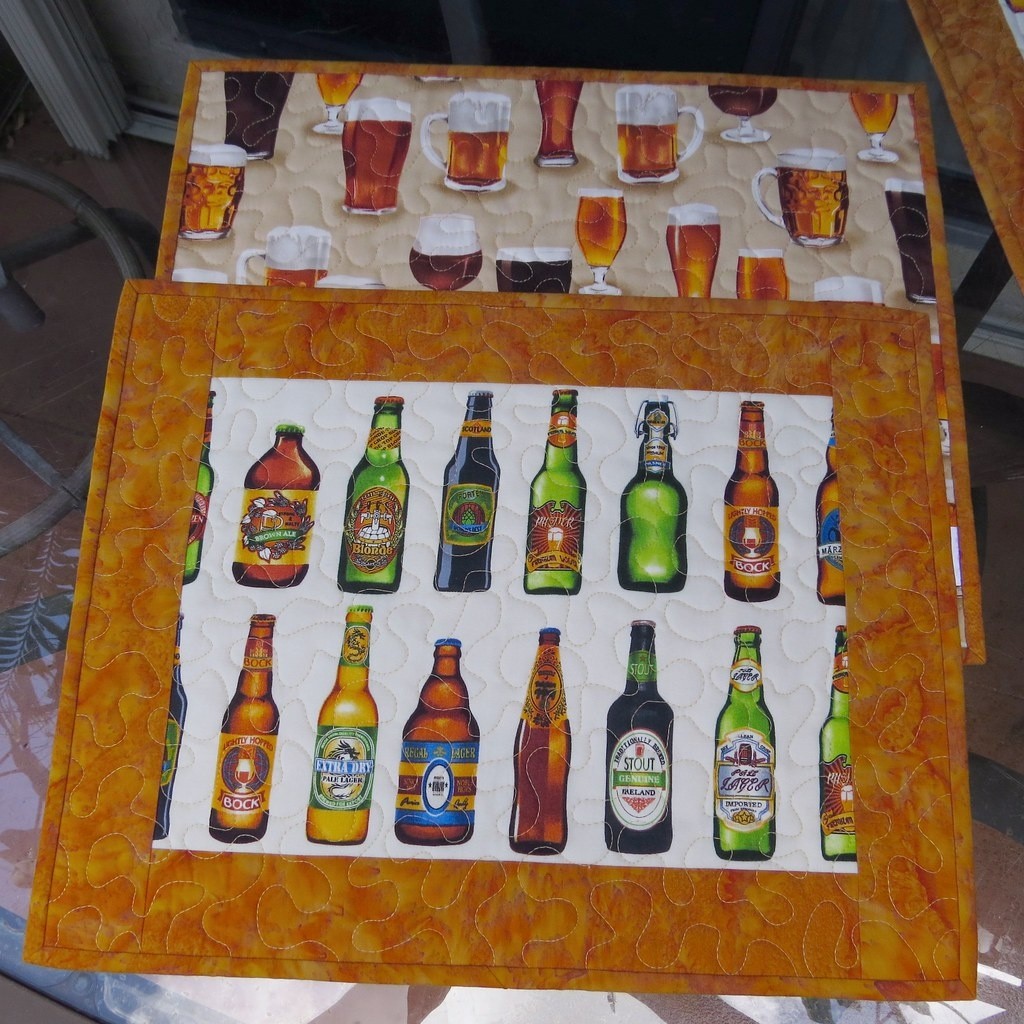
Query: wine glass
xmin=571 ymin=186 xmax=627 ymax=296
xmin=849 ymin=90 xmax=899 ymax=164
xmin=707 ymin=85 xmax=779 ymax=144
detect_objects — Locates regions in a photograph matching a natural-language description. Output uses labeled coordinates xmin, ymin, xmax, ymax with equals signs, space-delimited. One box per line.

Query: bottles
xmin=151 ymin=385 xmax=854 ymax=861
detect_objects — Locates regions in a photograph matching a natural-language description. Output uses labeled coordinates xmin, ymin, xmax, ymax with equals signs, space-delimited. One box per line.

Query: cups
xmin=175 ymin=66 xmax=939 ymax=309
